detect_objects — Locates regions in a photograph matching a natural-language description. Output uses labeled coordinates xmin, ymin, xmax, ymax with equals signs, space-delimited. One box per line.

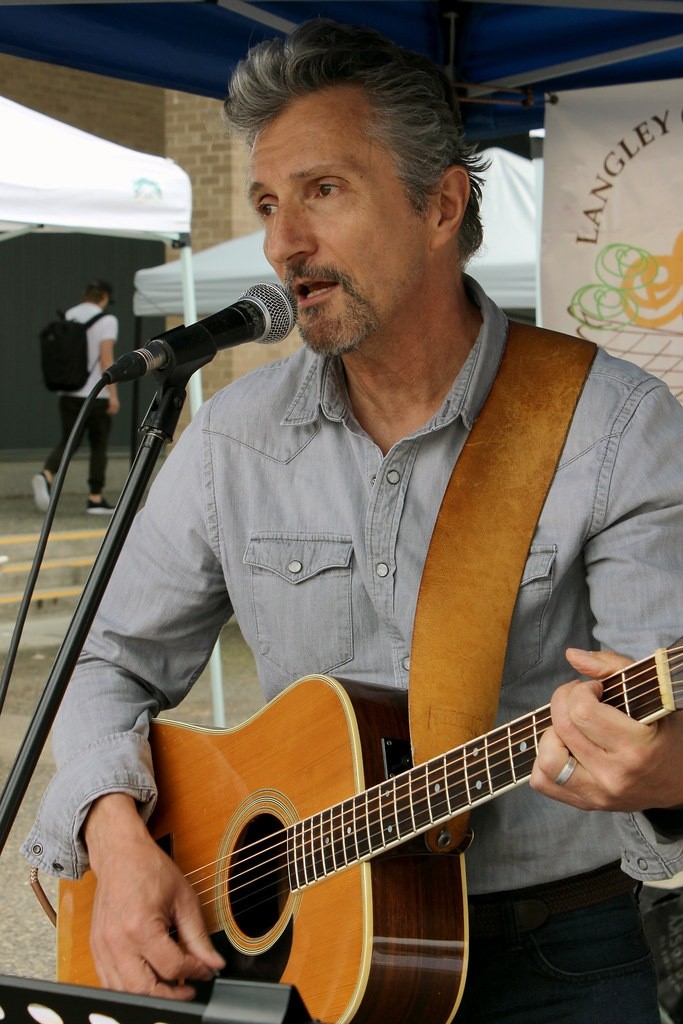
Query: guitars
xmin=55 ymin=637 xmax=683 ymax=1024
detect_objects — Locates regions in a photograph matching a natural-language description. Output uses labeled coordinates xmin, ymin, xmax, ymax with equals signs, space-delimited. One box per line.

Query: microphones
xmin=101 ymin=282 xmax=298 ymax=385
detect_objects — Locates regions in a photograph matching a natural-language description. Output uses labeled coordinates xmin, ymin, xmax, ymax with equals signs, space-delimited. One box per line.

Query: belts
xmin=469 ymin=860 xmax=641 ymax=937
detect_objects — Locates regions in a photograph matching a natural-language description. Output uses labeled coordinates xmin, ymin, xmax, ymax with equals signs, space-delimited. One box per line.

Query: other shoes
xmin=86 ymin=498 xmax=116 ymax=514
xmin=32 ymin=472 xmax=51 ymax=512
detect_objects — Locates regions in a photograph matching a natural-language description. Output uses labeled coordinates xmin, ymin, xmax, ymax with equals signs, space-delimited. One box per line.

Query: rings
xmin=555 ymin=756 xmax=578 ymax=786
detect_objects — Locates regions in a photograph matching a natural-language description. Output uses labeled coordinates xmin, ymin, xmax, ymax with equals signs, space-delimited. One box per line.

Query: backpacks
xmin=39 ymin=309 xmax=109 ymax=392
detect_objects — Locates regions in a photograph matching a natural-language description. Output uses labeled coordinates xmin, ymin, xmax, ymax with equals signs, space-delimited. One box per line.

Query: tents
xmin=0 ymin=0 xmax=683 ymax=141
xmin=0 ymin=92 xmax=226 ymax=731
xmin=132 ymin=147 xmax=545 ymax=319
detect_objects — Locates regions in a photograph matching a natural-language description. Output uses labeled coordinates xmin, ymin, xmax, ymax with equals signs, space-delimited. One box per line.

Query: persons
xmin=31 ymin=275 xmax=118 ymax=515
xmin=22 ymin=14 xmax=683 ymax=1024
